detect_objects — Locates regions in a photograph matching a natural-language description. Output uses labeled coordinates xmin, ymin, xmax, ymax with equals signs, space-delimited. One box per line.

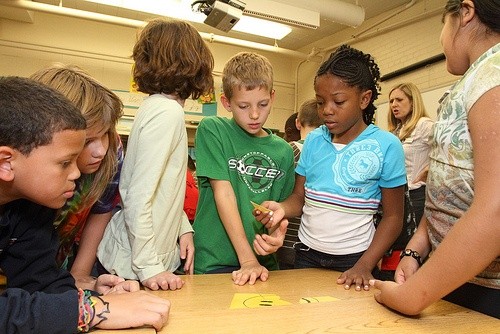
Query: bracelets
xmin=399 ymin=249 xmax=422 ymax=266
xmin=76 ymin=287 xmax=95 ymax=332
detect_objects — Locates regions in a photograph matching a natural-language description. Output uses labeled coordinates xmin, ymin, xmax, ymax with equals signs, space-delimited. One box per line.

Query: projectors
xmin=204 ymin=0 xmax=242 ymax=34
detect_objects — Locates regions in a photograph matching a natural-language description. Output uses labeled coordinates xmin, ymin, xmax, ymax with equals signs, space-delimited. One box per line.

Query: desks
xmin=86 ymin=268 xmax=500 ymax=334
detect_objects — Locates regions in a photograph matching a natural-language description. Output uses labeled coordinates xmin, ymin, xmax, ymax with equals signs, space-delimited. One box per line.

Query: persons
xmin=183 ymin=154 xmax=199 ymax=225
xmin=27 ymin=62 xmax=141 ymax=296
xmin=90 ymin=18 xmax=215 ymax=291
xmin=0 ymin=76 xmax=170 ymax=334
xmin=281 ymin=112 xmax=301 ymax=143
xmin=386 ymin=82 xmax=435 ymax=245
xmin=192 ymin=51 xmax=296 ymax=286
xmin=369 ymin=0 xmax=500 ymax=320
xmin=276 ymin=99 xmax=323 ymax=270
xmin=251 ymin=44 xmax=407 ymax=292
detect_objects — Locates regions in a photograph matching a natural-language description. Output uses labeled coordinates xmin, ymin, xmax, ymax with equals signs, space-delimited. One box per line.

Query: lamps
xmin=86 ymin=0 xmax=292 ymax=40
